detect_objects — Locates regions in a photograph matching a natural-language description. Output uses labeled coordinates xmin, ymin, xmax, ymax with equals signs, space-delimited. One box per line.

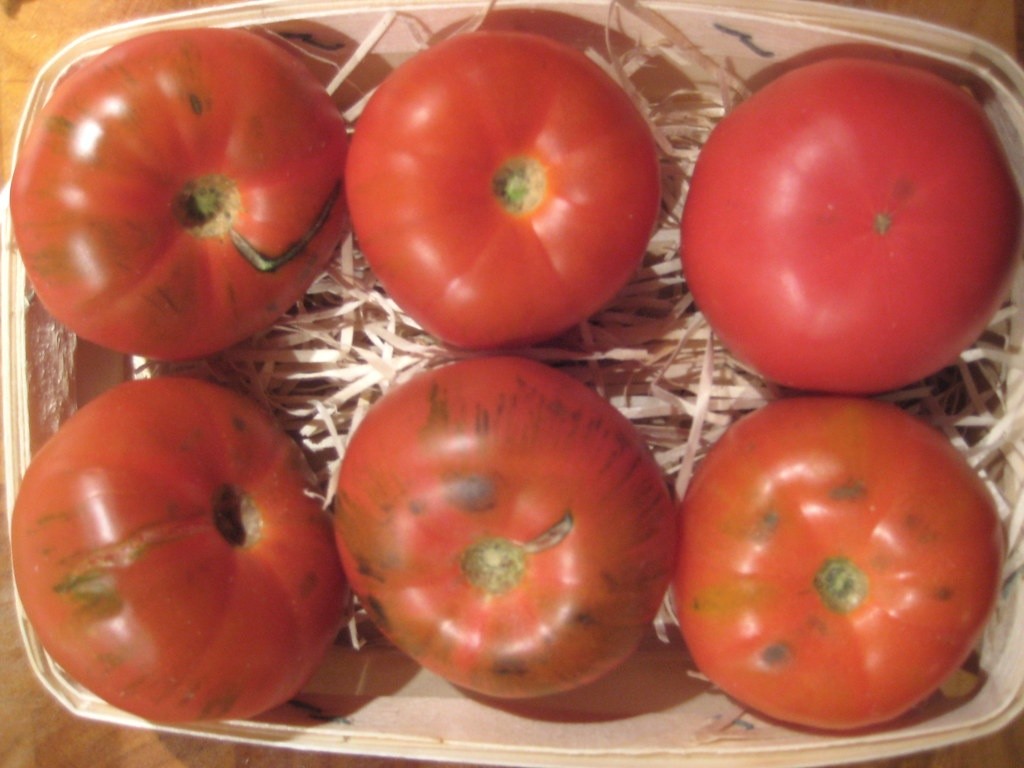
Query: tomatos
xmin=9 ymin=29 xmax=347 ymax=361
xmin=681 ymin=61 xmax=1020 ymax=396
xmin=674 ymin=397 xmax=1001 ymax=730
xmin=9 ymin=375 xmax=343 ymax=725
xmin=330 ymin=355 xmax=675 ymax=701
xmin=346 ymin=31 xmax=661 ymax=348
